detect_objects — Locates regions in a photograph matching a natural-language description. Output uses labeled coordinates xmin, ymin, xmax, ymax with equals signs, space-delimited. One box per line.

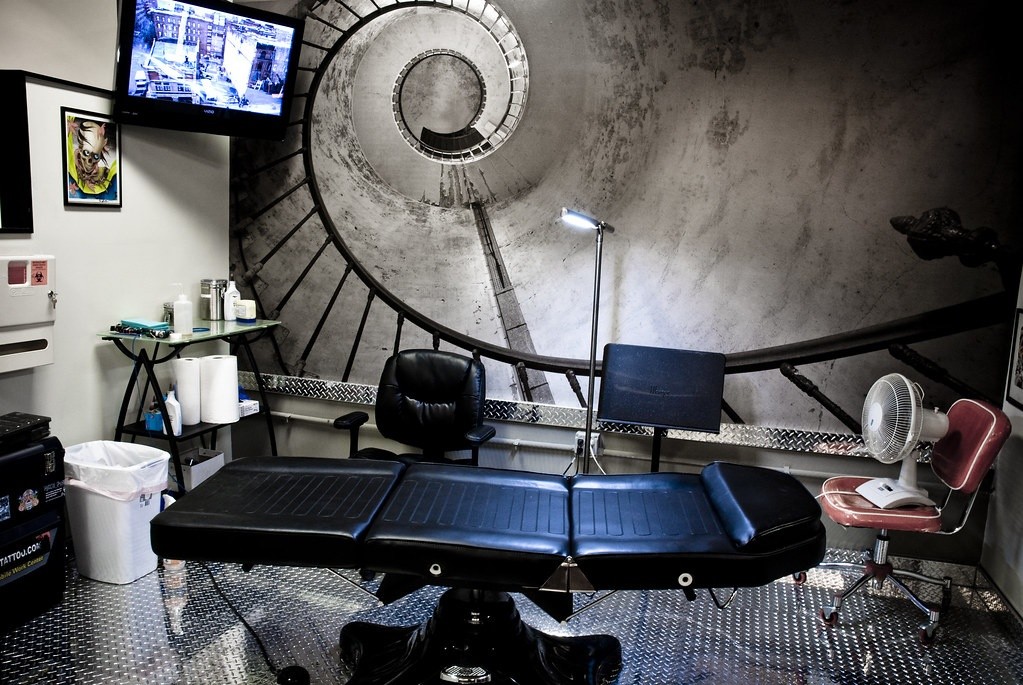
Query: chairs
xmin=335 ymin=349 xmax=495 ymax=466
xmin=791 ymin=398 xmax=1011 ymax=642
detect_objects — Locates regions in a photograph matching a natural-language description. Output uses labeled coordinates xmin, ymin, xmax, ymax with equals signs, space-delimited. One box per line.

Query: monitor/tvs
xmin=112 ymin=0 xmax=306 ymax=142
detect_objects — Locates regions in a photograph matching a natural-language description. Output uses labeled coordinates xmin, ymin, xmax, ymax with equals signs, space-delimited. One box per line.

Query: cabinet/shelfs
xmin=96 ymin=318 xmax=283 ymax=498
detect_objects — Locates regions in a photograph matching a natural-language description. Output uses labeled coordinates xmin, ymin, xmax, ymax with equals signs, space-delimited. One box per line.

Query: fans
xmin=855 ymin=371 xmax=949 ymax=510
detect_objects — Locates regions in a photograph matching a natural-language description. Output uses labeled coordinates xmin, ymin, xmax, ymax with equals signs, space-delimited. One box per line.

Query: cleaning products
xmin=162 ymin=388 xmax=182 ymax=437
xmin=161 ymin=494 xmax=187 ymax=572
xmin=223 ymin=280 xmax=257 ymax=324
xmin=163 ymin=569 xmax=189 ymax=636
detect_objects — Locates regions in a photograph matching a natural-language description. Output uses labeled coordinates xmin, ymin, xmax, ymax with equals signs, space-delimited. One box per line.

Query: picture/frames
xmin=59 ymin=106 xmax=123 ymax=208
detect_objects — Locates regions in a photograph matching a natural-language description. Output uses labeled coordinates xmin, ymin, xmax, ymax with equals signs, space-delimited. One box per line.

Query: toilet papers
xmin=171 ymin=357 xmax=201 ymax=426
xmin=199 ymin=354 xmax=240 ymax=425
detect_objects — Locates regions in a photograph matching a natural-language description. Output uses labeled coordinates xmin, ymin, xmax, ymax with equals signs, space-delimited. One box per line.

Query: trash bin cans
xmin=61 ymin=438 xmax=171 ymax=586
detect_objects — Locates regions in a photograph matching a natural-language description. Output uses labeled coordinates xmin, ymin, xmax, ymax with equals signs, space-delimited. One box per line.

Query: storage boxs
xmin=166 ymin=449 xmax=225 ymax=494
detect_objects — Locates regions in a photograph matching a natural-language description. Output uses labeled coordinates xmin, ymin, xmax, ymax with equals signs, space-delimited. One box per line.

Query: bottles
xmin=163 ymin=390 xmax=182 ymax=436
xmin=224 ymin=281 xmax=241 ymax=321
xmin=174 ymin=295 xmax=192 ymax=338
